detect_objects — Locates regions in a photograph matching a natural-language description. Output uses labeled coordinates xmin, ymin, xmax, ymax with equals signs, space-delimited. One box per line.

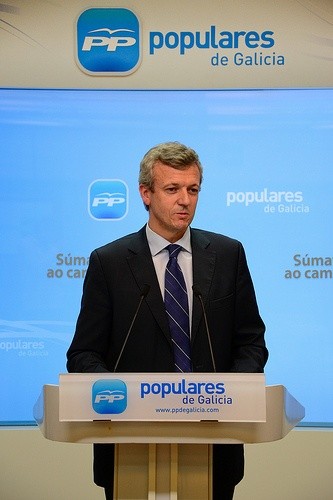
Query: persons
xmin=66 ymin=141 xmax=269 ymax=500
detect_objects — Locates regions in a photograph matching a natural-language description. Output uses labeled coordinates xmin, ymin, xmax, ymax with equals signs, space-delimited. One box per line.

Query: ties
xmin=164 ymin=244 xmax=191 ymax=373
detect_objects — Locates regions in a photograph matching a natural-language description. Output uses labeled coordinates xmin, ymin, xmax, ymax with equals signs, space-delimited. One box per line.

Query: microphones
xmin=113 ymin=283 xmax=151 ymax=372
xmin=192 ymin=285 xmax=216 ymax=373
